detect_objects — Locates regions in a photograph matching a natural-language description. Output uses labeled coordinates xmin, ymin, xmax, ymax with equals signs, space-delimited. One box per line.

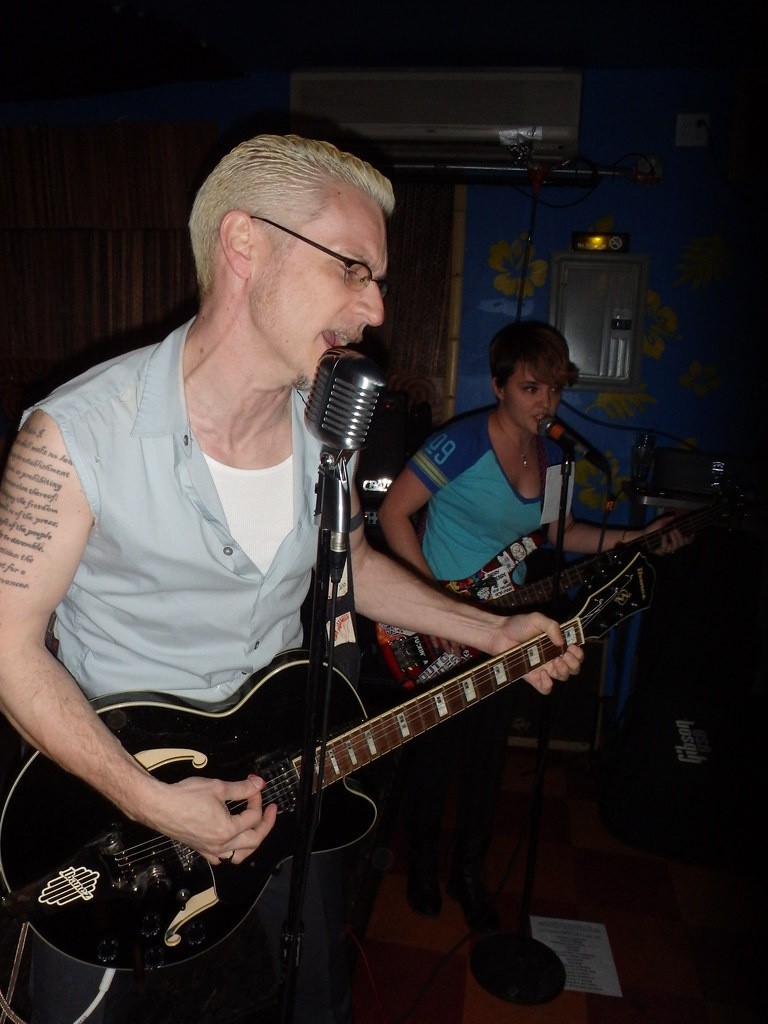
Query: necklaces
xmin=494 ymin=409 xmax=537 ymax=469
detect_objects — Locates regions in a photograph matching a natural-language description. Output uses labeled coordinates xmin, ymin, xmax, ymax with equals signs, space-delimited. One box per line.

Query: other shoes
xmin=448 ymin=882 xmax=500 ymax=934
xmin=405 ymin=863 xmax=443 ymax=919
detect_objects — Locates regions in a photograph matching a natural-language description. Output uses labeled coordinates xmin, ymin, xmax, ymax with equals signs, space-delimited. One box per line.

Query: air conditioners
xmin=289 ymin=66 xmax=584 ymax=164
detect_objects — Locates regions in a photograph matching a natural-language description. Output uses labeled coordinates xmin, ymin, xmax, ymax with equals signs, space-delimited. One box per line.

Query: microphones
xmin=538 ymin=417 xmax=600 ymax=468
xmin=305 ymin=346 xmax=386 ymax=471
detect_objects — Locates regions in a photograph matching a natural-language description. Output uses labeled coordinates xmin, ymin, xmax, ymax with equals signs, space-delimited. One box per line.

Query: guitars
xmin=377 ymin=475 xmax=768 ymax=692
xmin=0 ymin=547 xmax=662 ymax=973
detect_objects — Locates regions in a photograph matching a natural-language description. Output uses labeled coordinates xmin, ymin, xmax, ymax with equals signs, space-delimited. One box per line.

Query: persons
xmin=374 ymin=320 xmax=700 ymax=921
xmin=0 ymin=132 xmax=586 ymax=1023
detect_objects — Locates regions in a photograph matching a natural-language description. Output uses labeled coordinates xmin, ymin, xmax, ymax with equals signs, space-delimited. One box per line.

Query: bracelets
xmin=621 ymin=530 xmax=627 ymax=543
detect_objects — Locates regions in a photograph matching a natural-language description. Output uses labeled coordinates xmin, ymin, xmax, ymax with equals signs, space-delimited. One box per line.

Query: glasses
xmin=249 ymin=214 xmax=388 ymax=302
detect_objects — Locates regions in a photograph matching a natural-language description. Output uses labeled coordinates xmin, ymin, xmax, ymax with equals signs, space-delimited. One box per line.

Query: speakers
xmin=601 ymin=525 xmax=768 ymax=874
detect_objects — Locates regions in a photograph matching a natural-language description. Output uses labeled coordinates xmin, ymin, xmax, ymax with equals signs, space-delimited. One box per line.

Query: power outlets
xmin=675 ymin=113 xmax=709 ymax=149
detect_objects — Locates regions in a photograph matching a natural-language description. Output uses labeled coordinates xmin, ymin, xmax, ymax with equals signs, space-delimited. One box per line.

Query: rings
xmin=218 ymin=850 xmax=235 ymax=864
xmin=671 ymin=551 xmax=675 ymax=554
